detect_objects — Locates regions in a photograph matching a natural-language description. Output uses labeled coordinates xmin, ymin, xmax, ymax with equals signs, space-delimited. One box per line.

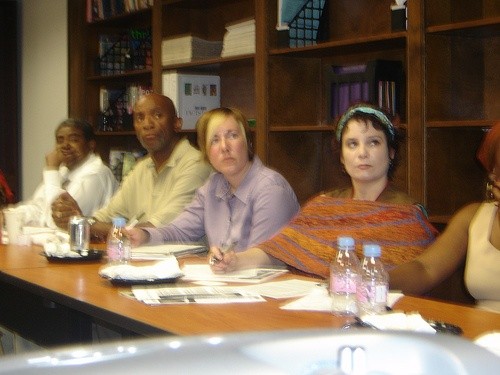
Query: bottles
xmin=358 ymin=244 xmax=390 ymax=315
xmin=3 ymin=208 xmax=27 ymax=247
xmin=329 ymin=236 xmax=362 ymax=317
xmin=107 ymin=218 xmax=133 ymax=270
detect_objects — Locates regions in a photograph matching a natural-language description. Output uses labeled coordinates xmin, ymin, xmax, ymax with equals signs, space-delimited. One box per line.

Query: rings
xmin=54 ymin=211 xmax=62 ymax=217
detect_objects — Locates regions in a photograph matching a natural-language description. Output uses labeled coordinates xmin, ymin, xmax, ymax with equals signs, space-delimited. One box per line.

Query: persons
xmin=124 ymin=106 xmax=300 ymax=254
xmin=387 ymin=123 xmax=500 ymax=312
xmin=51 ymin=93 xmax=215 ymax=239
xmin=0 ymin=118 xmax=121 ymax=228
xmin=209 ymin=103 xmax=443 ymax=281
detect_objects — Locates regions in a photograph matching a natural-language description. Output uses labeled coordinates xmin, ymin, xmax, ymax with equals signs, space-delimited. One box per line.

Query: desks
xmin=0 ymin=243 xmax=500 ymax=347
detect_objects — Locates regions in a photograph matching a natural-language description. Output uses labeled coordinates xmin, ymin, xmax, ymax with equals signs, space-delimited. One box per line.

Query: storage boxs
xmin=162 ymin=72 xmax=221 ymax=129
xmin=327 ymin=59 xmax=405 ymax=127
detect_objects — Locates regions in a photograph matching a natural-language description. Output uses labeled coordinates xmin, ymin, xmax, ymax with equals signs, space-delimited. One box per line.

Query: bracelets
xmin=88 ymin=216 xmax=98 ymax=226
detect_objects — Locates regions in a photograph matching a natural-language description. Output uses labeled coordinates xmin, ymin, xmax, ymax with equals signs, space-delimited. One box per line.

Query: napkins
xmin=102 ymin=253 xmax=181 ymax=279
xmin=43 ymin=241 xmax=82 ymax=258
xmin=360 ymin=312 xmax=436 ymax=334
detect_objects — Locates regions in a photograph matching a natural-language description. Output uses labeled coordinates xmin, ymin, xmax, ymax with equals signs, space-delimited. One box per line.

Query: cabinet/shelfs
xmin=67 ymin=0 xmax=500 ymax=301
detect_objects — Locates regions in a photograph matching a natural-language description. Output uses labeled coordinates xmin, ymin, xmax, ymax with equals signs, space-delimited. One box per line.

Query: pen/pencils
xmin=426 ymin=319 xmax=465 ymax=334
xmin=128 ymin=211 xmax=146 ymax=228
xmin=210 ymin=239 xmax=239 ymax=263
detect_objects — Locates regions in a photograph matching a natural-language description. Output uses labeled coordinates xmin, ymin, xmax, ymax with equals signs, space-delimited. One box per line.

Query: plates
xmin=37 ymin=248 xmax=106 ymax=263
xmin=97 ymin=266 xmax=185 ymax=282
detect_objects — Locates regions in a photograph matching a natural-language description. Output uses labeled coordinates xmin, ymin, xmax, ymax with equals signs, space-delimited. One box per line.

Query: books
xmin=98 ymin=29 xmax=152 ymax=77
xmin=160 ymin=35 xmax=219 ymax=65
xmin=161 ymin=72 xmax=221 ymax=130
xmin=86 ymin=0 xmax=153 ymax=23
xmin=221 ymin=19 xmax=256 ymax=58
xmin=96 ymin=84 xmax=153 ymax=131
xmin=106 ymin=147 xmax=145 ymax=180
